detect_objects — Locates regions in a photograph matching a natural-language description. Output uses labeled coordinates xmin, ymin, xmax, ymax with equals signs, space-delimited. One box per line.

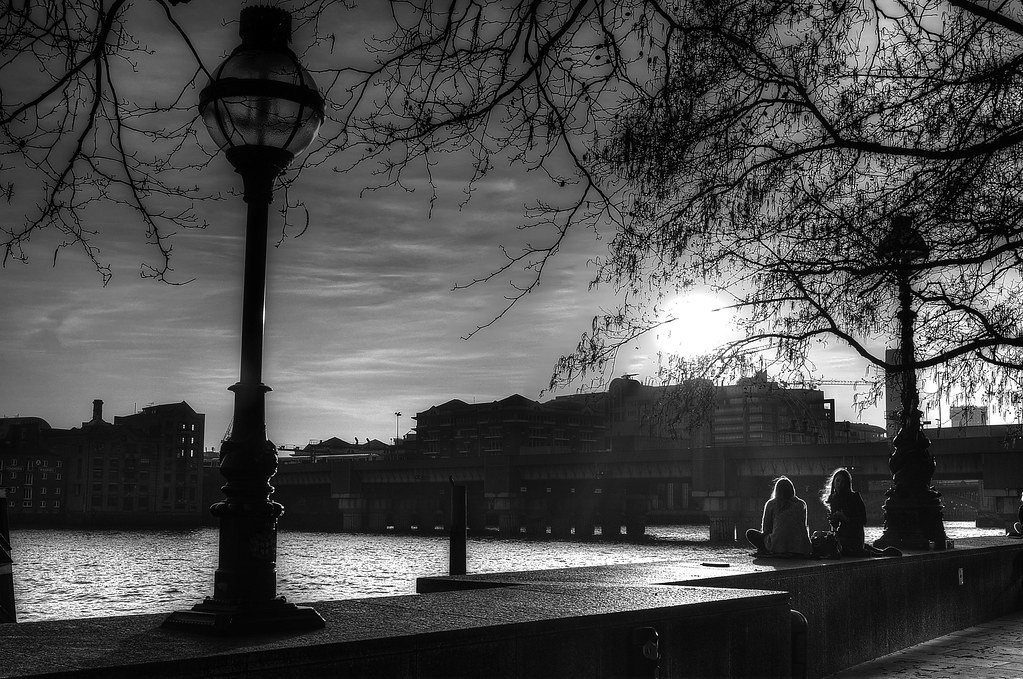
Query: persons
xmin=1014 ymin=492 xmax=1023 ymax=534
xmin=813 ymin=470 xmax=867 ymax=553
xmin=746 ymin=479 xmax=813 ymax=556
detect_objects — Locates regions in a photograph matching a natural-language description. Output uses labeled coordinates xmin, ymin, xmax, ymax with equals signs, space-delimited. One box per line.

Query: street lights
xmin=171 ymin=36 xmax=331 ymax=639
xmin=872 ymin=228 xmax=955 ymax=553
